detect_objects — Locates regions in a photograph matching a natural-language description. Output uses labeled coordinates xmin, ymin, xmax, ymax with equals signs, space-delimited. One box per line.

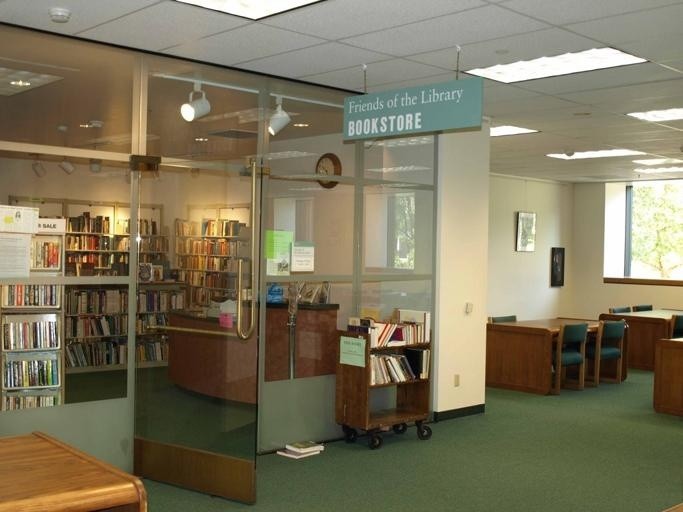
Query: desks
xmin=651 ymin=338 xmax=682 ymax=416
xmin=600 ymin=310 xmax=678 ymax=370
xmin=487 ymin=315 xmax=605 ymax=392
xmin=0 ymin=431 xmax=147 ymax=512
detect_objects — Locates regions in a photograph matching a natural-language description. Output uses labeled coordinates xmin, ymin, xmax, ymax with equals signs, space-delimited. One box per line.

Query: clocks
xmin=314 ymin=153 xmax=341 ymax=189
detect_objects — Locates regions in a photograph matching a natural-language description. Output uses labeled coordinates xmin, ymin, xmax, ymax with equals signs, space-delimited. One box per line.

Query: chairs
xmin=609 ymin=305 xmax=631 ymax=313
xmin=550 ymin=321 xmax=588 ymax=396
xmin=632 ymin=304 xmax=652 ymax=312
xmin=488 ymin=315 xmax=516 ymax=325
xmin=583 ymin=318 xmax=626 ymax=388
xmin=667 ymin=314 xmax=682 ymax=339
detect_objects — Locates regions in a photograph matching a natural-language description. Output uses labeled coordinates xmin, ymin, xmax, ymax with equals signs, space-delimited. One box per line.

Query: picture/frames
xmin=514 ymin=211 xmax=536 ymax=253
xmin=549 ymin=247 xmax=564 ymax=286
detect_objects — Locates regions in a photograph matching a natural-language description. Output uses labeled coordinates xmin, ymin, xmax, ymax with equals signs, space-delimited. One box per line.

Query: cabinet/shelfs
xmin=3 ymin=229 xmax=255 ymax=408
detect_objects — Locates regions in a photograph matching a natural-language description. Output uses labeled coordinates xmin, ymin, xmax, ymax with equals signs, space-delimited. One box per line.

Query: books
xmin=64 ymin=211 xmax=131 ymax=276
xmin=139 ymin=219 xmax=178 ymax=282
xmin=1 ymin=395 xmax=55 ymax=412
xmin=64 ymin=340 xmax=127 ymax=369
xmin=1 ymin=284 xmax=59 ymax=307
xmin=345 ymin=306 xmax=429 ymax=387
xmin=2 ymin=320 xmax=59 ymax=348
xmin=136 ymin=289 xmax=186 ymax=362
xmin=173 ymin=218 xmax=247 ymax=313
xmin=64 ymin=287 xmax=128 ymax=336
xmin=2 ymin=360 xmax=59 ymax=388
xmin=274 ymin=440 xmax=325 ymax=461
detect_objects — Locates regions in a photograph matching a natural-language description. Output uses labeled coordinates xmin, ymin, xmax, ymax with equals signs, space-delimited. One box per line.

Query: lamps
xmin=30 ymin=119 xmax=103 ymax=177
xmin=151 ymin=72 xmax=343 ymax=137
